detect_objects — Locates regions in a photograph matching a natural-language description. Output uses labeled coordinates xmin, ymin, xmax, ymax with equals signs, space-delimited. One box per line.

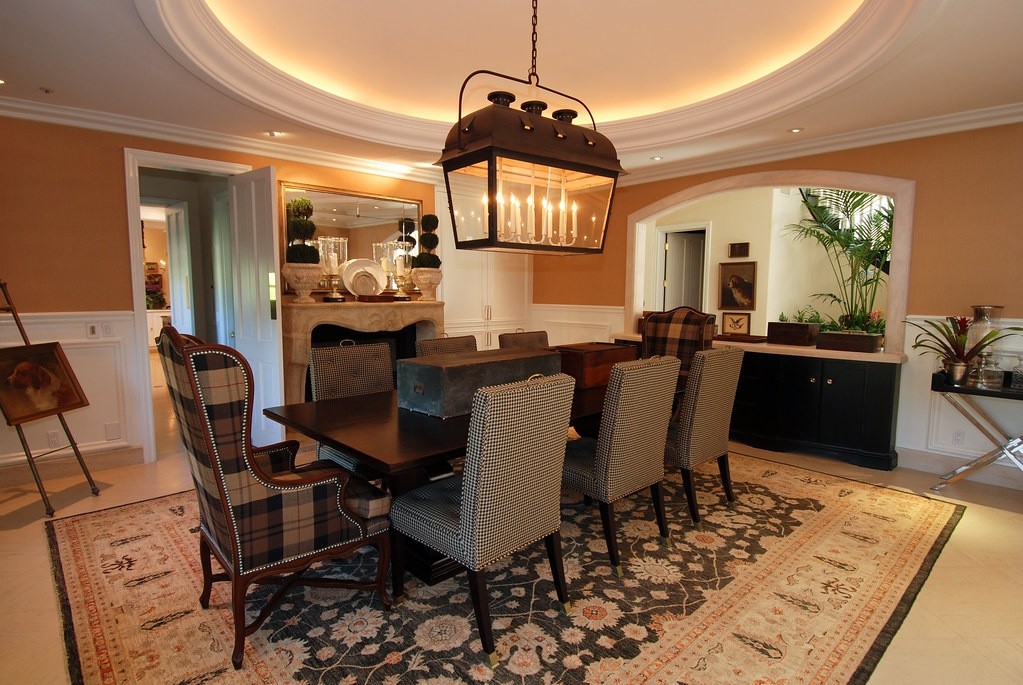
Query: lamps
xmin=433 ymin=1 xmax=630 ymax=256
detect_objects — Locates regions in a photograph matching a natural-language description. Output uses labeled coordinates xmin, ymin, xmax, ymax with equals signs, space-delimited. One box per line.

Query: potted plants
xmin=412 ymin=214 xmax=442 ymax=301
xmin=392 ymin=218 xmax=417 ymax=292
xmin=283 ymin=195 xmax=322 ymax=304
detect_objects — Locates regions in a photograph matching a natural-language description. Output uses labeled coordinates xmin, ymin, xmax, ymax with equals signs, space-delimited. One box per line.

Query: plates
xmin=352 ymin=271 xmax=378 ymax=296
xmin=342 ymin=259 xmax=388 ymax=296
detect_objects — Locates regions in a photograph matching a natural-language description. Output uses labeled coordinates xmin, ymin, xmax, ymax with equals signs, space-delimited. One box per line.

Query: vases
xmin=942 ymin=359 xmax=974 ymax=387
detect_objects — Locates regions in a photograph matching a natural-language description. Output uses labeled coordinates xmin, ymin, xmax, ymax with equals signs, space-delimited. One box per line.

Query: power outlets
xmin=47 ymin=431 xmax=62 ymax=448
xmin=86 ymin=321 xmax=99 ymax=340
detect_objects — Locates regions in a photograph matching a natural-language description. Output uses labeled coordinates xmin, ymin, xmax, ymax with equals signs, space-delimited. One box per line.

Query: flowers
xmin=905 ymin=314 xmax=1008 ymax=362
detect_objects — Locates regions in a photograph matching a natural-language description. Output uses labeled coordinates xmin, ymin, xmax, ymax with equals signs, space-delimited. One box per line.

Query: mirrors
xmin=278 ymin=180 xmax=425 ymax=293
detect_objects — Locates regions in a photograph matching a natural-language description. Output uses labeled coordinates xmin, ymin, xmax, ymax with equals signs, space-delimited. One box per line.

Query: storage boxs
xmin=544 ymin=342 xmax=638 ymax=389
xmin=395 ymin=346 xmax=563 ymax=417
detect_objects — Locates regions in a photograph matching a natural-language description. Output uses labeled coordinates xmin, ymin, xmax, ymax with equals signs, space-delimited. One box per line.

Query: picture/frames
xmin=722 ymin=310 xmax=749 ymax=335
xmin=717 ymin=262 xmax=757 ymax=311
xmin=144 ymin=262 xmax=158 ymax=274
xmin=0 ymin=341 xmax=90 ymax=427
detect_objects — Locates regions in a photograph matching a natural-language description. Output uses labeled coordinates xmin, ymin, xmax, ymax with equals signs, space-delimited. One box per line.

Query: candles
xmin=330 ymin=252 xmax=337 ymax=275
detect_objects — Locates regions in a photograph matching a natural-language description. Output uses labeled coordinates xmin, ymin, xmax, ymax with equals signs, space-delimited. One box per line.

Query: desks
xmin=264 ymin=375 xmax=691 ymax=587
xmin=928 ymin=368 xmax=1023 ymax=490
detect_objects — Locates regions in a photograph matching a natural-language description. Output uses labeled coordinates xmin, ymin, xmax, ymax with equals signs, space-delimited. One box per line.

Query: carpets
xmin=41 ymin=452 xmax=966 ymax=685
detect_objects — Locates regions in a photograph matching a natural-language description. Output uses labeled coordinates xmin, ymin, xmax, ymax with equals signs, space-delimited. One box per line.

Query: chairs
xmin=308 ymin=342 xmax=396 ymax=490
xmin=392 ymin=373 xmax=573 ymax=668
xmin=641 ymin=306 xmax=716 ymax=371
xmin=154 ymin=323 xmax=392 ymax=670
xmin=417 ymin=336 xmax=477 ymax=357
xmin=498 ymin=331 xmax=549 ymax=348
xmin=664 ymin=345 xmax=745 ymax=520
xmin=558 ymin=356 xmax=681 ymax=566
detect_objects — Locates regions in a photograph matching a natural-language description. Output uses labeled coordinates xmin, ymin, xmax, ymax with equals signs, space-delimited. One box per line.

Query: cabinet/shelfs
xmin=147 ymin=313 xmax=162 ymax=347
xmin=615 ymin=339 xmax=901 ymax=472
